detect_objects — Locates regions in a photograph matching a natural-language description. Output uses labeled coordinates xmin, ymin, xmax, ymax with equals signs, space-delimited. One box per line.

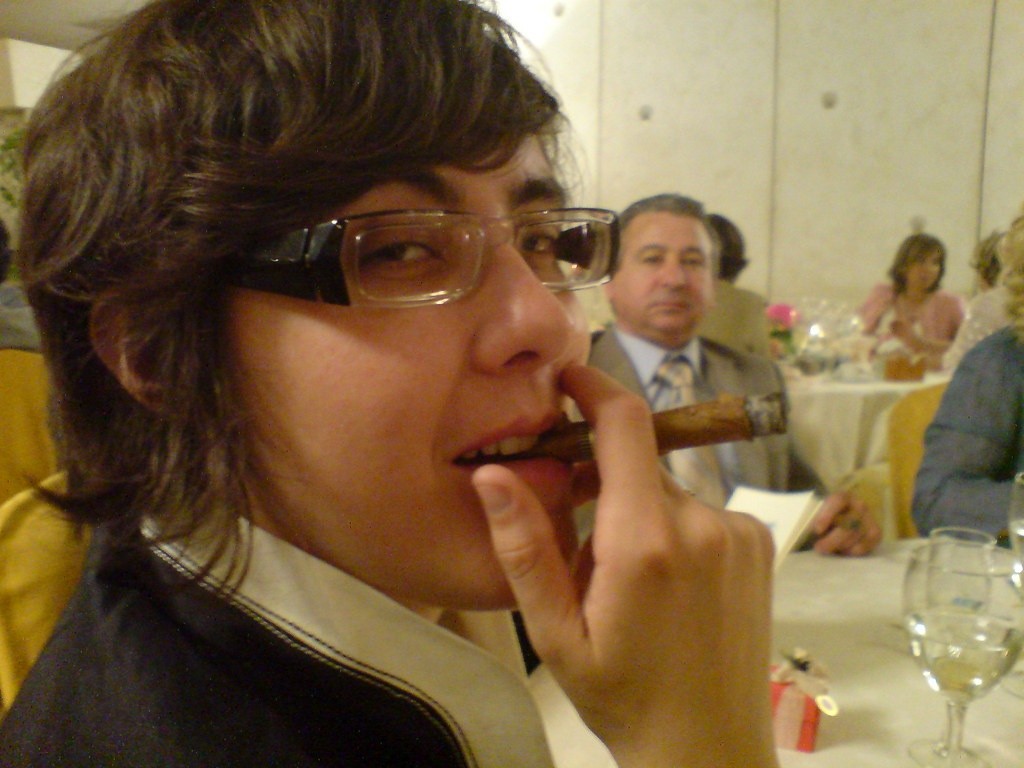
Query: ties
xmin=654 ymin=362 xmax=724 ymax=513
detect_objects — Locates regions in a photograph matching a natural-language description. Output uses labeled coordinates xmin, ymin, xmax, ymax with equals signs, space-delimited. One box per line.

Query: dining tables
xmin=784 ymin=368 xmax=951 ymax=541
xmin=525 ymin=530 xmax=1022 ymax=768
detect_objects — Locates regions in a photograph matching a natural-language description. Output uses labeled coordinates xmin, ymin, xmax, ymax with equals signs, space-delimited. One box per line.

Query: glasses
xmin=211 ymin=208 xmax=620 ymax=308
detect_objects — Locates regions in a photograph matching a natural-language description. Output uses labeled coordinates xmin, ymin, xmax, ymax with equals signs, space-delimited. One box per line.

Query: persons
xmin=703 ymin=213 xmax=823 ymax=494
xmin=912 ymin=217 xmax=1024 ymax=550
xmin=856 ymin=234 xmax=966 ymax=371
xmin=588 ymin=192 xmax=884 ymax=558
xmin=0 ymin=0 xmax=780 ymax=768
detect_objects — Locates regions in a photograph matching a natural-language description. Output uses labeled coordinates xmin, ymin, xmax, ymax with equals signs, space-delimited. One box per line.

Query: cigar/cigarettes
xmin=834 ymin=514 xmax=860 ymax=529
xmin=541 ymin=392 xmax=792 ymax=464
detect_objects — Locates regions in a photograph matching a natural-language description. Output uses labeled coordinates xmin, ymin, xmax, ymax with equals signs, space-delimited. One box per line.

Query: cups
xmin=1008 ymin=473 xmax=1024 ymax=557
xmin=922 ymin=528 xmax=998 ymax=613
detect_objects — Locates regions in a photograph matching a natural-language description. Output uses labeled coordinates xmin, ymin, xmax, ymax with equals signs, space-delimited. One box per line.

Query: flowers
xmin=766 ymin=303 xmax=797 ymax=352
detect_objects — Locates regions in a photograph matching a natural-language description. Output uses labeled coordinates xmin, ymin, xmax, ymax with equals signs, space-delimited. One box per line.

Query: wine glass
xmin=900 ymin=541 xmax=1024 ymax=768
